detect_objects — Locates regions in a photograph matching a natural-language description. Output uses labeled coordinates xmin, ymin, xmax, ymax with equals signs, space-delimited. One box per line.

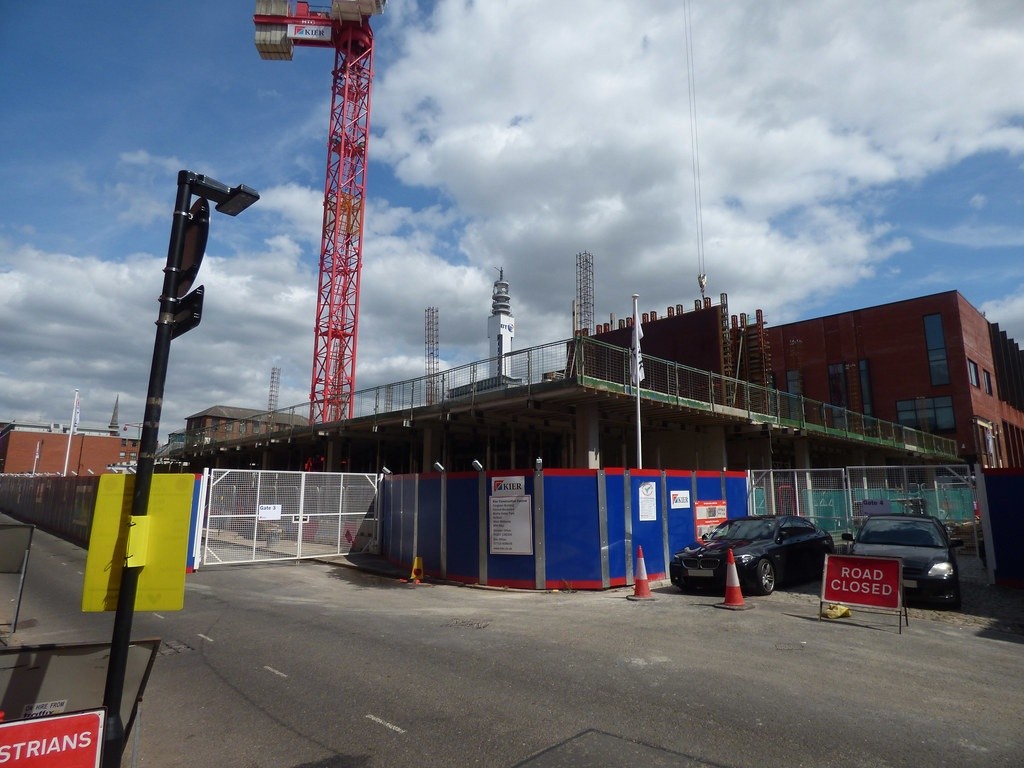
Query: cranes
xmin=251 ymin=1 xmax=388 ymax=423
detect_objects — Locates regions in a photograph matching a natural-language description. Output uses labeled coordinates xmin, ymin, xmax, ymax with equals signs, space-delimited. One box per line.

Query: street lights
xmin=97 ymin=169 xmax=260 ymax=768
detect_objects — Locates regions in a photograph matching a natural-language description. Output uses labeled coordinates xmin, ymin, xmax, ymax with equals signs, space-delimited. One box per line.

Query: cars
xmin=669 ymin=514 xmax=834 ymax=596
xmin=841 ymin=514 xmax=964 ymax=612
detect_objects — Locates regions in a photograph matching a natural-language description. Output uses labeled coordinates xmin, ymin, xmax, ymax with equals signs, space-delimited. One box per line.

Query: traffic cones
xmin=625 ymin=545 xmax=660 ymax=602
xmin=712 ymin=548 xmax=755 ymax=611
